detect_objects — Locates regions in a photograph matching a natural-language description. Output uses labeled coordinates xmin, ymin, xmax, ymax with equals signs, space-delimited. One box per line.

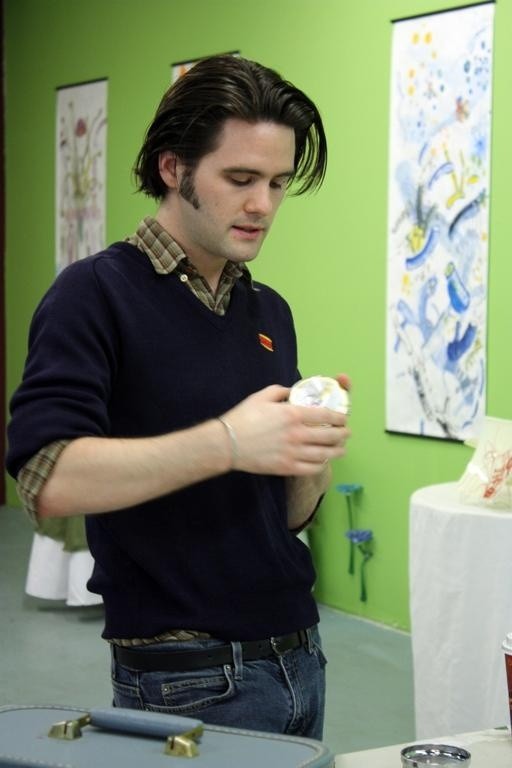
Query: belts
xmin=110 ymin=628 xmax=310 ymax=673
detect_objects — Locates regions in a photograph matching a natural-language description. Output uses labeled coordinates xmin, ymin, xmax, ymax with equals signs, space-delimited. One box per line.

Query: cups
xmin=501 ymin=634 xmax=512 ymax=728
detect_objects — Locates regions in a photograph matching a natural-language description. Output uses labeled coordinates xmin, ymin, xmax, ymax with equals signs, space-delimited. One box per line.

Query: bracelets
xmin=219 ymin=415 xmax=239 ymax=478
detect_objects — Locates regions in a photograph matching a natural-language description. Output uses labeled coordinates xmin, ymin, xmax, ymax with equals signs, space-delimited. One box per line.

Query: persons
xmin=3 ymin=48 xmax=356 ymax=744
